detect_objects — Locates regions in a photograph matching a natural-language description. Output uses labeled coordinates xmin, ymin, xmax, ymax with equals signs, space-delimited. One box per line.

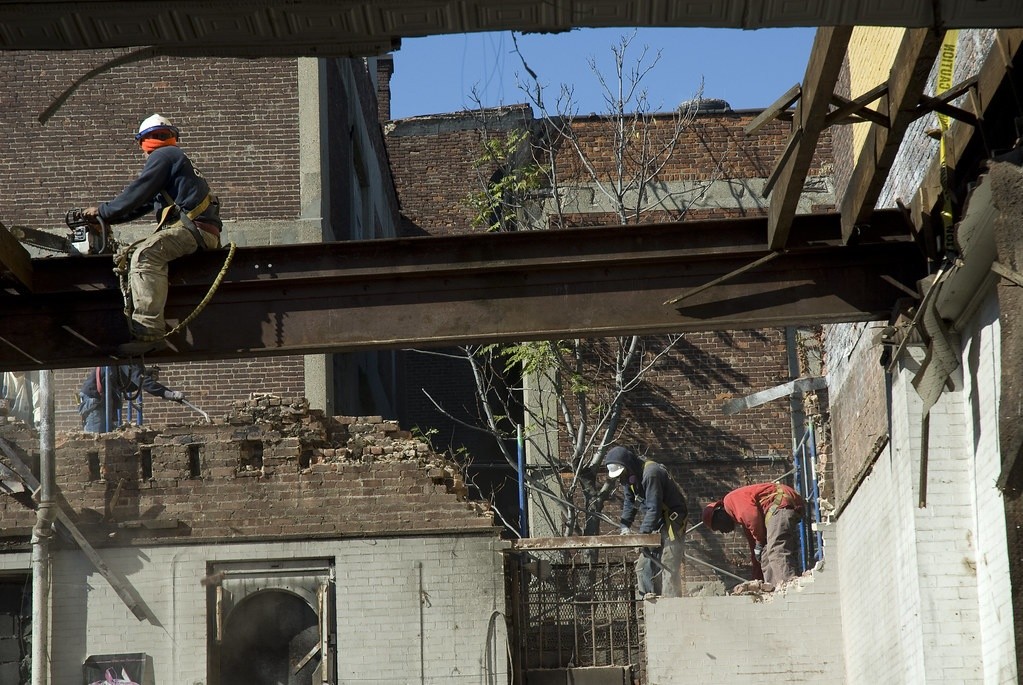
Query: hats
xmin=606 ymin=463 xmax=625 ymax=478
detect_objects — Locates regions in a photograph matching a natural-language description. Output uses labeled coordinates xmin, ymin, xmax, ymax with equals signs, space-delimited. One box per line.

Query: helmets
xmin=135 ymin=113 xmax=180 ymax=141
xmin=702 ymin=500 xmax=724 ymax=529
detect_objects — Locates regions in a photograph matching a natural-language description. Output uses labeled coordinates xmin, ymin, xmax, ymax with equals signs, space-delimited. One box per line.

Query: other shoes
xmin=118 ymin=334 xmax=168 ymax=355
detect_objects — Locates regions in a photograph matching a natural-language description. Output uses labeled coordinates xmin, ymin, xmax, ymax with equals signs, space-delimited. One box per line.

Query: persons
xmin=79 ymin=112 xmax=222 ymax=354
xmin=701 ymin=483 xmax=805 ymax=584
xmin=605 ymin=447 xmax=689 ymax=598
xmin=77 ymin=365 xmax=189 ymax=434
xmin=0 ymin=370 xmax=41 ymax=433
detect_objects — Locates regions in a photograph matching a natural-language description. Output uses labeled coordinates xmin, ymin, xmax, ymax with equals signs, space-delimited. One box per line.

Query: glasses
xmin=146 ymin=133 xmax=174 ymax=141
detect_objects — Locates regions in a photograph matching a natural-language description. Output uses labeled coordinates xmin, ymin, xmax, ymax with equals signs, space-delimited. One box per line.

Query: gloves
xmin=755 ymin=544 xmax=764 ymax=561
xmin=620 ymin=527 xmax=631 ymax=535
xmin=163 ymin=389 xmax=185 ymax=402
